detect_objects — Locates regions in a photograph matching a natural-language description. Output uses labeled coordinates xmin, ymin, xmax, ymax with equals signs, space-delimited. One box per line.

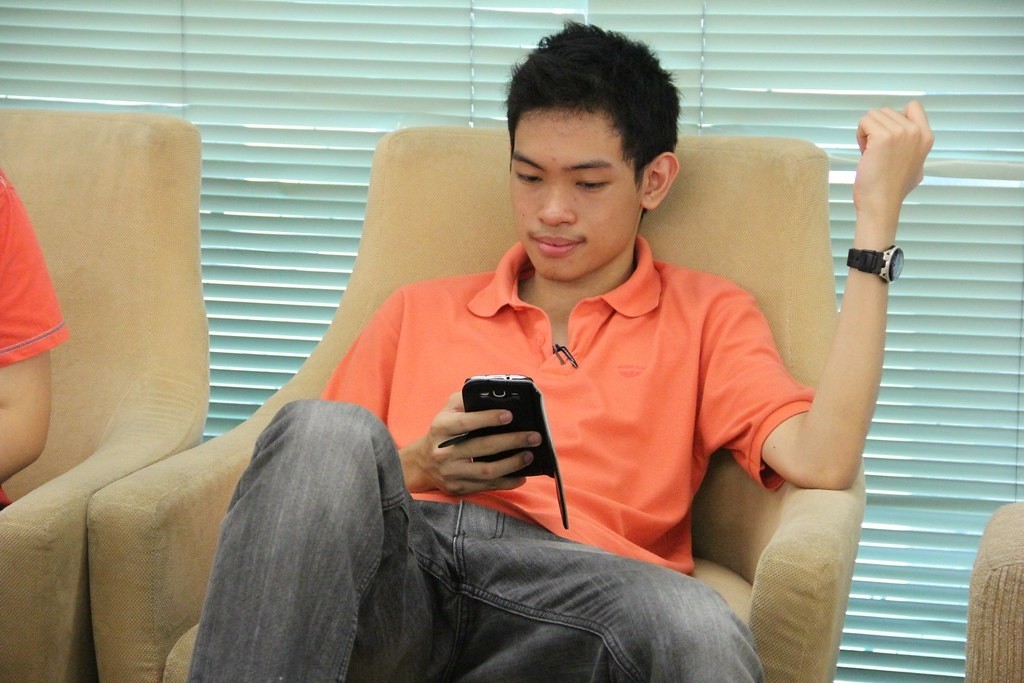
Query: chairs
xmin=1 ymin=111 xmax=204 ymax=683
xmin=89 ymin=126 xmax=863 ymax=683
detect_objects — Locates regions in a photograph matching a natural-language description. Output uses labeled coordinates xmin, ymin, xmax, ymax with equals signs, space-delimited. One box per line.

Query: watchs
xmin=844 ymin=245 xmax=908 ymax=283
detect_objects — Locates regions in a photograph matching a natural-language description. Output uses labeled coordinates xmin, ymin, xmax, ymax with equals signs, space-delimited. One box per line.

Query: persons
xmin=0 ymin=168 xmax=69 ymax=530
xmin=181 ymin=20 xmax=932 ymax=683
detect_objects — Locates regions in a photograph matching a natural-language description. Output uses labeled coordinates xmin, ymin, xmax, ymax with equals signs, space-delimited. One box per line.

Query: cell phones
xmin=461 ymin=374 xmax=554 ymax=478
